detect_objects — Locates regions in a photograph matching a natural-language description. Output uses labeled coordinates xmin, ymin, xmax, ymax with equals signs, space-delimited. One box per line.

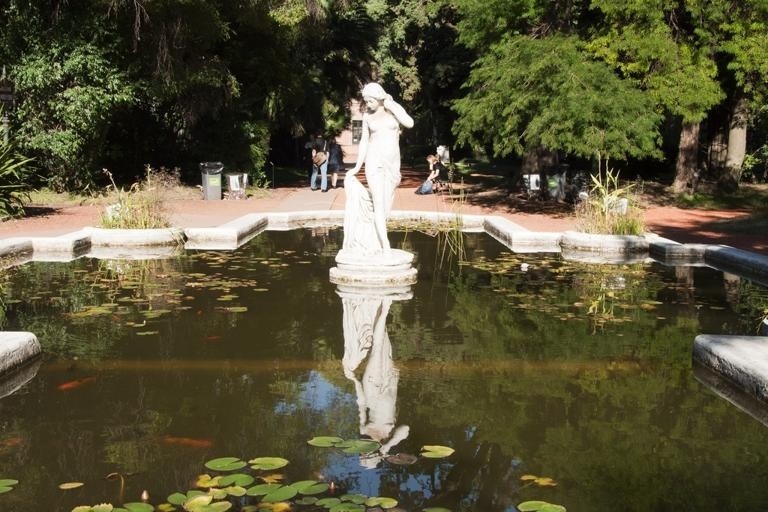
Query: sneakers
xmin=311 ymin=184 xmax=337 ymax=192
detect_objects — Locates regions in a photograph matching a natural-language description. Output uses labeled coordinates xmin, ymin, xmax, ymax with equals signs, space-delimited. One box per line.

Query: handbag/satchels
xmin=414 ymin=179 xmax=433 ymax=194
xmin=312 ymin=151 xmax=326 ymax=167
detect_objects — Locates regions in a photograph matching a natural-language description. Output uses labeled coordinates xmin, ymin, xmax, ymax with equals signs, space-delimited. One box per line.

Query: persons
xmin=346 ymin=82 xmax=414 ymax=252
xmin=342 ymin=296 xmax=412 ymax=471
xmin=310 ymin=130 xmax=328 ymax=192
xmin=327 ymin=138 xmax=343 ymax=188
xmin=416 ymin=154 xmax=448 ymax=195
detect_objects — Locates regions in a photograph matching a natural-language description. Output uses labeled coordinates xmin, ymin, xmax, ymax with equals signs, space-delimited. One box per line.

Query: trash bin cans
xmin=540 ymin=164 xmax=569 ymax=201
xmin=200 ymin=162 xmax=225 ymax=200
